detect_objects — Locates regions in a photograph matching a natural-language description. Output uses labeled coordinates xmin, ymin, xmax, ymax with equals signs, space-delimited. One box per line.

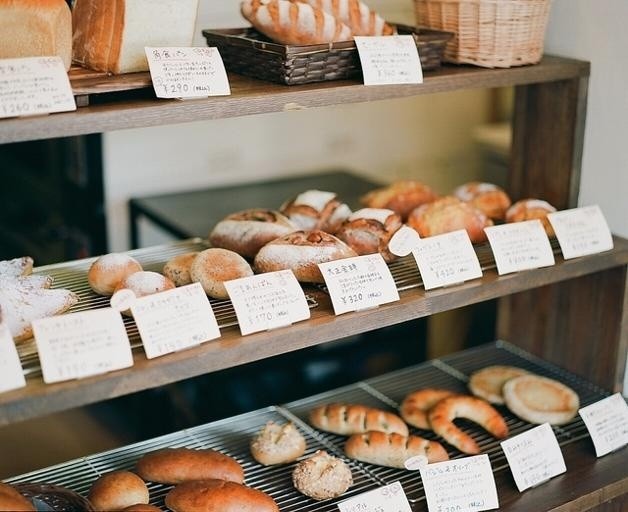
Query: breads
xmin=344 ymin=431 xmax=448 ymax=470
xmin=505 ymin=197 xmax=559 ymax=237
xmin=138 ymin=448 xmax=244 ymax=484
xmin=210 ymin=208 xmax=300 ymax=259
xmin=190 ymin=247 xmax=254 ymax=301
xmin=358 ymin=180 xmax=439 ymax=219
xmin=502 ymin=374 xmax=580 ymax=425
xmin=0 ymin=256 xmax=80 ymax=345
xmin=0 ymin=478 xmax=36 ymax=512
xmin=87 ymin=253 xmax=143 ymax=296
xmin=453 ymin=181 xmax=512 ymax=220
xmin=88 ymin=471 xmax=150 ymax=512
xmin=304 ymin=0 xmax=396 ymax=36
xmin=70 ymin=1 xmax=200 ymax=74
xmin=337 ymin=207 xmax=405 ymax=264
xmin=240 ymin=0 xmax=355 ymax=45
xmin=164 ymin=480 xmax=280 ymax=512
xmin=0 ymin=0 xmax=74 ymax=74
xmin=292 ymin=449 xmax=353 ymax=501
xmin=112 ymin=271 xmax=176 ymax=316
xmin=406 ymin=196 xmax=494 ymax=243
xmin=254 ymin=229 xmax=360 ymax=284
xmin=426 ymin=395 xmax=508 ymax=454
xmin=163 ymin=251 xmax=201 ymax=286
xmin=250 ymin=420 xmax=306 ymax=466
xmin=119 ymin=504 xmax=163 ymax=512
xmin=279 ymin=188 xmax=352 ymax=235
xmin=309 ymin=405 xmax=409 ymax=437
xmin=468 ymin=365 xmax=537 ymax=404
xmin=400 ymin=387 xmax=458 ymax=430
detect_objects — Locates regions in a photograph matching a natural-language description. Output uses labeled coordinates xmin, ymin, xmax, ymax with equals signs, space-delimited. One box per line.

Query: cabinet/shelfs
xmin=0 ymin=45 xmax=627 ymax=512
xmin=121 ymin=168 xmax=432 ymax=441
